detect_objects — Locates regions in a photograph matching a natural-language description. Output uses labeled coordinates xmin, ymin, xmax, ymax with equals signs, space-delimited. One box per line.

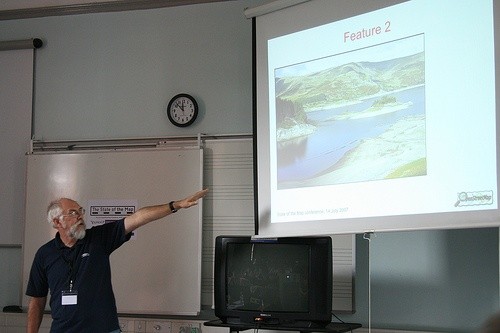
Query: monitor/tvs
xmin=214 ymin=236 xmax=332 ymax=329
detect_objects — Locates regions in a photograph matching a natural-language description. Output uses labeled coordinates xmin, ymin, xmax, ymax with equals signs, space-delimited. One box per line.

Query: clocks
xmin=166 ymin=94 xmax=198 ymax=126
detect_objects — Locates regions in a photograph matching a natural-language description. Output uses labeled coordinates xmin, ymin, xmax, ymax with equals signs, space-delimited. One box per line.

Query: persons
xmin=25 ymin=188 xmax=209 ymax=333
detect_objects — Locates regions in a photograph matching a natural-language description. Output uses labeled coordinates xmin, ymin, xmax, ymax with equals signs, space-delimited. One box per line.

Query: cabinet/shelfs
xmin=0 ymin=311 xmax=211 ymax=333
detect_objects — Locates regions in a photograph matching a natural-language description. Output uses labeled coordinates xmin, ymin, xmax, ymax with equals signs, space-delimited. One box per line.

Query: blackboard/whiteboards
xmin=18 ymin=145 xmax=204 ymax=319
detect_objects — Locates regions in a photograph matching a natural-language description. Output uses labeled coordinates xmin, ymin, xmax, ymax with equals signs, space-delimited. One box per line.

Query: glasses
xmin=62 ymin=208 xmax=86 ymax=218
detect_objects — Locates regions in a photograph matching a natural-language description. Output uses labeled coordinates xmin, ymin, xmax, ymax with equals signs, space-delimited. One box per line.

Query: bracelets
xmin=169 ymin=201 xmax=178 ymax=213
